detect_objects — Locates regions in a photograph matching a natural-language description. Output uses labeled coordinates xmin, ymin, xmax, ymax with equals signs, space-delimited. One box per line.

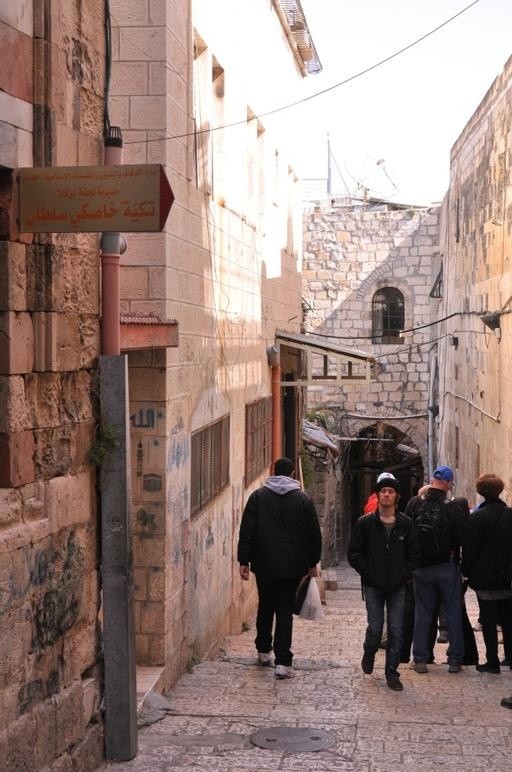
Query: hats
xmin=376 ymin=472 xmax=396 ymax=482
xmin=375 ymin=479 xmax=401 ymax=492
xmin=432 ymin=466 xmax=454 ymax=482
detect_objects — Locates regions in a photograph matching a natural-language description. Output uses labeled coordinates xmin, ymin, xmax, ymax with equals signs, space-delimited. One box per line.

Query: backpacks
xmin=414 ymin=502 xmax=449 ymax=554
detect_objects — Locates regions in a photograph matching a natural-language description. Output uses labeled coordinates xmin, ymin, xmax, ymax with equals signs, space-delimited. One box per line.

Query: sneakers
xmin=274 ymin=663 xmax=295 ymax=679
xmin=501 ymin=696 xmax=512 ymax=709
xmin=499 ymin=658 xmax=511 ymax=666
xmin=257 ymin=651 xmax=271 ymax=667
xmin=448 ymin=663 xmax=461 ymax=673
xmin=437 ymin=631 xmax=448 ymax=643
xmin=443 ymin=655 xmax=478 ymax=665
xmin=476 ymin=660 xmax=501 ymax=674
xmin=410 ymin=661 xmax=428 ymax=674
xmin=361 ymin=656 xmax=374 ymax=674
xmin=386 ymin=675 xmax=403 ymax=691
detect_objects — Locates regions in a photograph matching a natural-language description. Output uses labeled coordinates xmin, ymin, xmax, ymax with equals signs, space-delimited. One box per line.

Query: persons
xmin=345 ymin=463 xmax=511 ymax=695
xmin=236 ymin=455 xmax=323 ymax=680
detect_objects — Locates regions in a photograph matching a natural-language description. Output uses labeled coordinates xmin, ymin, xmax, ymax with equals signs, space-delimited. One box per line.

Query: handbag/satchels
xmin=460 ymin=557 xmax=484 ymax=591
